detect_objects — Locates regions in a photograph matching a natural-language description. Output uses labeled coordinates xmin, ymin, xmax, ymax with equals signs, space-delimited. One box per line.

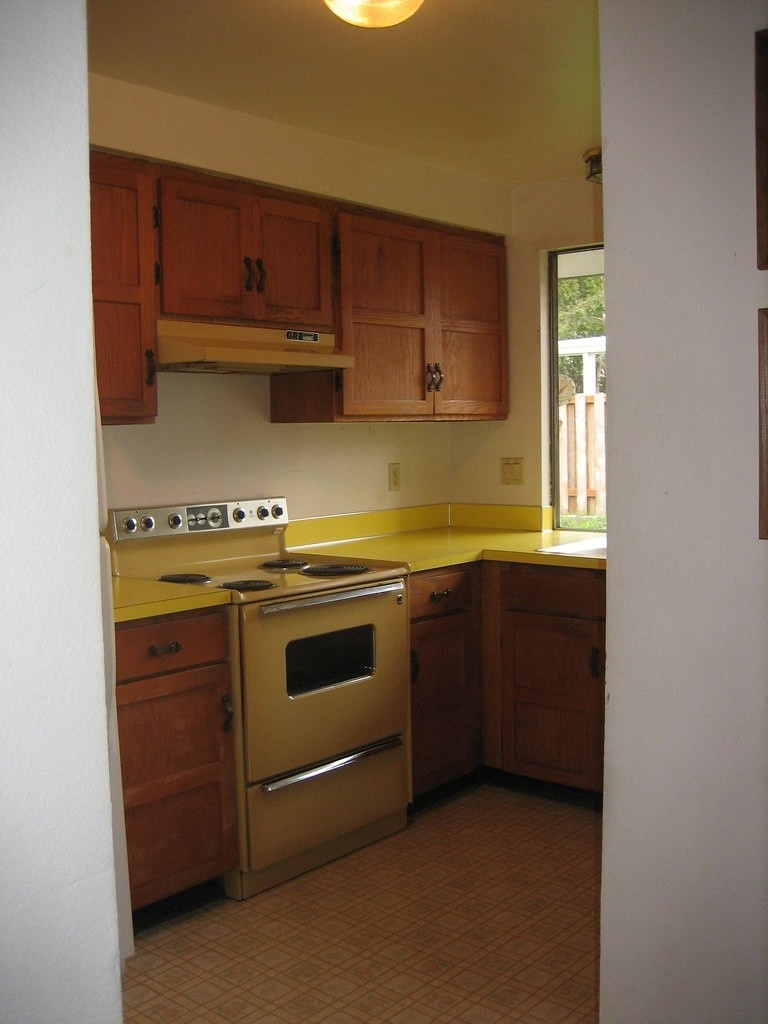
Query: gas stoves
xmin=107 ymin=496 xmax=409 ymax=604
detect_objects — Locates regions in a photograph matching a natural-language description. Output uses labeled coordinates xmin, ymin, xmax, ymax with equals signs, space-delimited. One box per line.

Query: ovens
xmin=230 ymin=577 xmax=410 ymax=873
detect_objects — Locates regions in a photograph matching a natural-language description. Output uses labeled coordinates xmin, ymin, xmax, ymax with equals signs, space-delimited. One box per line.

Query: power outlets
xmin=388 ymin=463 xmax=401 ymax=491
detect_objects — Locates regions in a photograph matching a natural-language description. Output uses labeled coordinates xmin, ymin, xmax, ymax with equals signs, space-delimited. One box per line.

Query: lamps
xmin=582 ymin=147 xmax=602 ymax=186
xmin=324 ymin=0 xmax=424 ymax=29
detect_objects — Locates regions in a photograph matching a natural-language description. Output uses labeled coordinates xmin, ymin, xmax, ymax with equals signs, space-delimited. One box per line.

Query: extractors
xmin=154 ymin=318 xmax=356 ymax=376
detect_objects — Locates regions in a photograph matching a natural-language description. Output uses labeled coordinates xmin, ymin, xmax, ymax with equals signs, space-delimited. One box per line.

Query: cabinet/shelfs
xmin=409 ymin=558 xmax=608 ymax=824
xmin=112 ymin=576 xmax=241 ymax=935
xmin=90 ymin=139 xmax=512 ymax=425
